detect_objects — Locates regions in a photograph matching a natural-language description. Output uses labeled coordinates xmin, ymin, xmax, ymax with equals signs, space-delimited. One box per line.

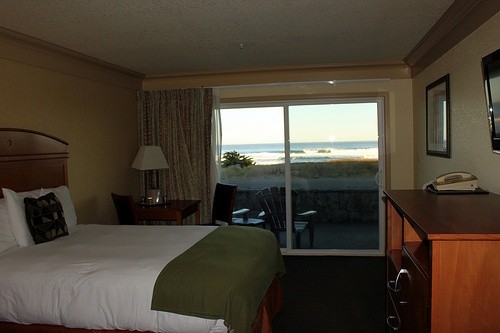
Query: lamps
xmin=132 ymin=144 xmax=169 ymax=205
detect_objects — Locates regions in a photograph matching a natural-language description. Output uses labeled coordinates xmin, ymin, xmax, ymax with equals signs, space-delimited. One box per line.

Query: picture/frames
xmin=482 ymin=49 xmax=500 ymax=151
xmin=425 ymin=73 xmax=450 ymax=159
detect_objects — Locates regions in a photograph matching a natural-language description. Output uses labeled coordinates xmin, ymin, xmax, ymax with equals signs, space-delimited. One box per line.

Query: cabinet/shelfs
xmin=382 ymin=189 xmax=500 ymax=333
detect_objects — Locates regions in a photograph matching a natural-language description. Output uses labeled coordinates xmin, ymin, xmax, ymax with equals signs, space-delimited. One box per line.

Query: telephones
xmin=431 ymin=172 xmax=480 ymax=191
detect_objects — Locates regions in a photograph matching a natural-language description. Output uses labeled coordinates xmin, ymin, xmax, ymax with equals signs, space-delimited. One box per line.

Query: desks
xmin=232 ymin=218 xmax=265 ymax=229
xmin=136 ymin=200 xmax=201 ymax=225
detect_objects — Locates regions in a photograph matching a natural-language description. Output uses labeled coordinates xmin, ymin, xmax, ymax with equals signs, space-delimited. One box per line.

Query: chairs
xmin=111 ymin=193 xmax=140 ymax=225
xmin=198 ymin=183 xmax=238 ymax=226
xmin=255 ymin=187 xmax=318 ymax=249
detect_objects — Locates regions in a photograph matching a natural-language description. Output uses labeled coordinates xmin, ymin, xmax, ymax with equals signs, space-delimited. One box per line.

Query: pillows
xmin=0 ymin=185 xmax=77 ymax=254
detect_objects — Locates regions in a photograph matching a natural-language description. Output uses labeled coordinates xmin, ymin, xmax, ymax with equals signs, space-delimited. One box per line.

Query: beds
xmin=0 ymin=127 xmax=286 ymax=333
